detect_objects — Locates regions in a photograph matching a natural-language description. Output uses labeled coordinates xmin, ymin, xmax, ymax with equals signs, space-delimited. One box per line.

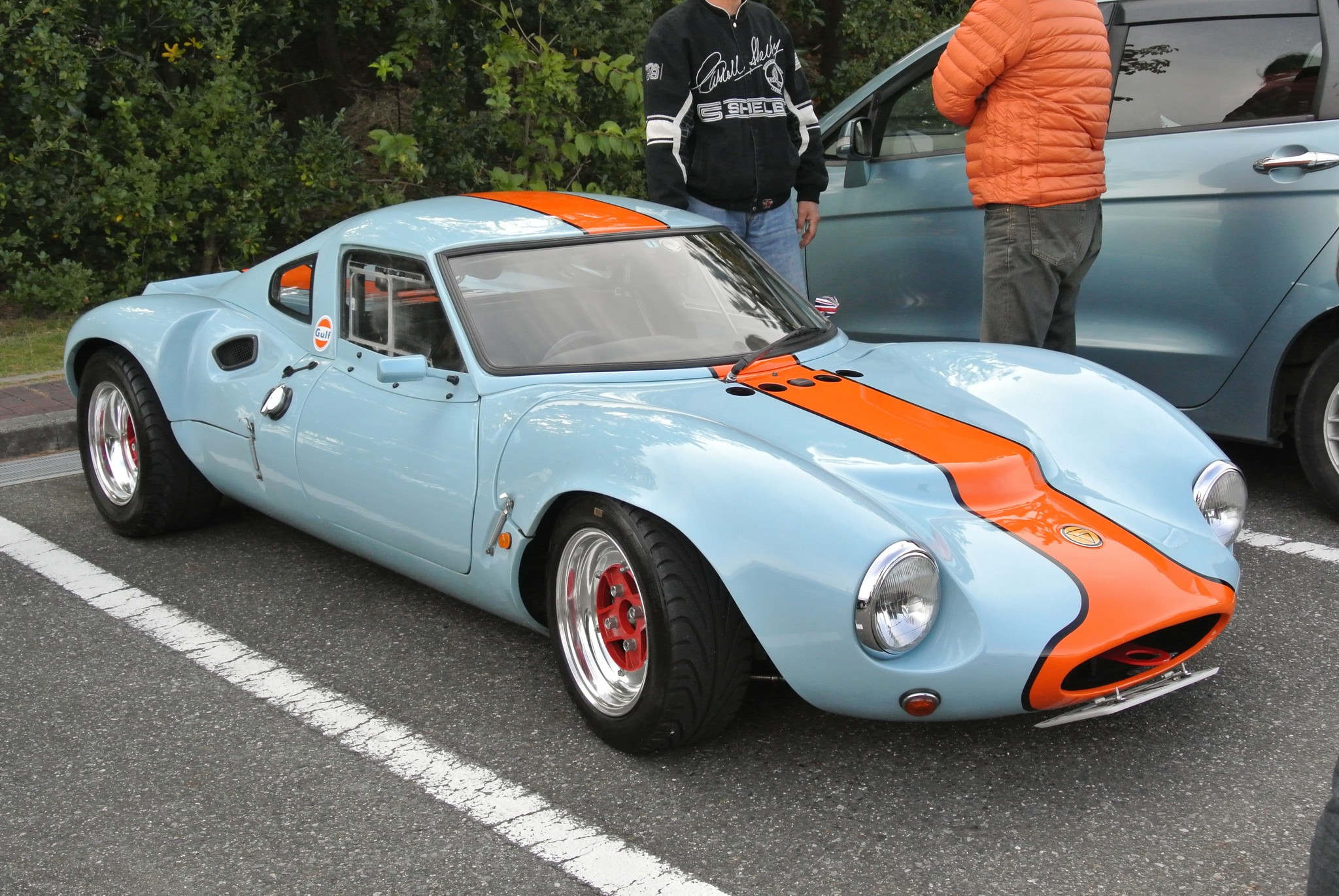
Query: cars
xmin=801 ymin=0 xmax=1338 ymax=518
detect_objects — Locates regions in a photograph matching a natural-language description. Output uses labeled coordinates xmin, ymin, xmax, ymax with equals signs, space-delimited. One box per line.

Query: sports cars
xmin=62 ymin=191 xmax=1248 ymax=760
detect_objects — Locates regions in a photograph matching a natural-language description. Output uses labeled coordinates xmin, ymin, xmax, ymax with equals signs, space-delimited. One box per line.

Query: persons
xmin=641 ymin=0 xmax=830 ymax=303
xmin=930 ymin=0 xmax=1114 ymax=358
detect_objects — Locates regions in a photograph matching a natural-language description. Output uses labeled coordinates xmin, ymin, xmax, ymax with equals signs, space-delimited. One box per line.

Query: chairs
xmin=431 ymin=295 xmax=548 ymax=371
